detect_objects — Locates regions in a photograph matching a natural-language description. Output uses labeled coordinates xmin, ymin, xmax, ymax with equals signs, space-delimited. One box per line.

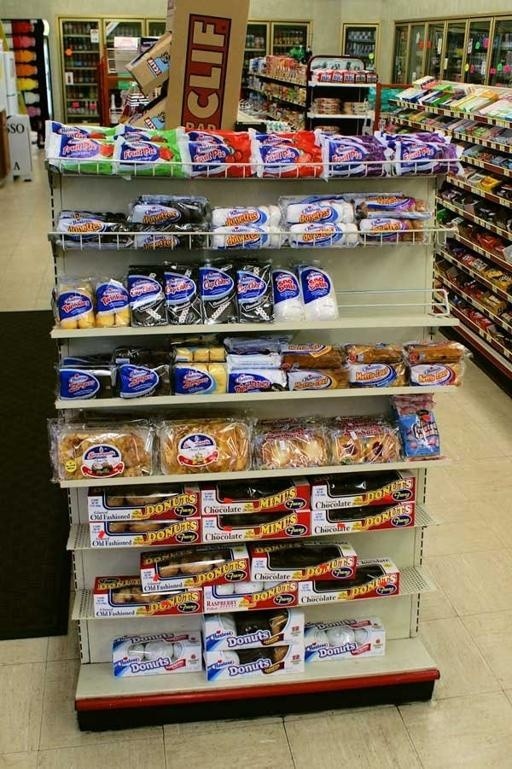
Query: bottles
xmin=241 ymin=30 xmax=266 ymax=87
xmin=62 ymin=21 xmax=101 ymax=117
xmin=106 ymin=21 xmax=166 ymax=39
xmin=274 ymin=29 xmax=306 ymax=56
xmin=346 ymin=30 xmax=375 ymax=71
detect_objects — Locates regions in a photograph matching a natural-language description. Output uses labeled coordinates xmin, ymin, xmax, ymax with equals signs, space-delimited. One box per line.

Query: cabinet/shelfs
xmin=434 ymin=134 xmax=511 ymax=383
xmin=61 ymin=15 xmax=171 ymax=130
xmin=44 ymin=120 xmax=458 ymax=733
xmin=235 ymin=12 xmax=512 ymax=133
